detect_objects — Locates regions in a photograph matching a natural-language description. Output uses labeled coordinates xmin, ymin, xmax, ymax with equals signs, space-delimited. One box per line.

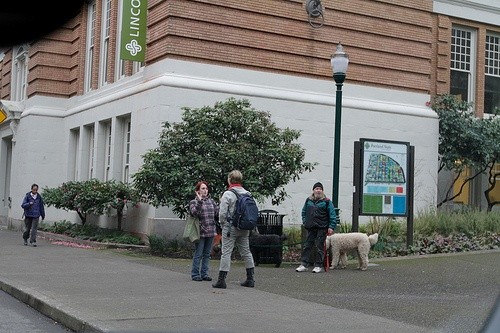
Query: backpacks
xmin=226 ymin=189 xmax=258 ymax=230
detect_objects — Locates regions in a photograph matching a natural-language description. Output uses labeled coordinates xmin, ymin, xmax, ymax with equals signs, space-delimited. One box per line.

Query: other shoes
xmin=312 ymin=266 xmax=323 ymax=273
xmin=24 ymin=239 xmax=28 ymax=245
xmin=30 ymin=241 xmax=37 ymax=247
xmin=192 ymin=276 xmax=202 ymax=281
xmin=202 ymin=277 xmax=212 ymax=281
xmin=295 ymin=265 xmax=309 ymax=272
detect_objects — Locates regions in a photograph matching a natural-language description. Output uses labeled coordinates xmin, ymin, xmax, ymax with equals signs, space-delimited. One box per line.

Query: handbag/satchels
xmin=184 ymin=217 xmax=201 ymax=243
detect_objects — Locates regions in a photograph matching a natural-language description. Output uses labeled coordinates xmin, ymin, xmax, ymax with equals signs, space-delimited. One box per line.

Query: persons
xmin=190 ymin=181 xmax=220 ymax=281
xmin=296 ymin=182 xmax=336 ymax=273
xmin=215 ymin=170 xmax=255 ymax=288
xmin=21 ymin=184 xmax=46 ymax=247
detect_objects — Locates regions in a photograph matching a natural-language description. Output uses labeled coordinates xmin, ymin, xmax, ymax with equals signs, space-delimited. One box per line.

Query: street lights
xmin=328 ymin=39 xmax=350 ymax=224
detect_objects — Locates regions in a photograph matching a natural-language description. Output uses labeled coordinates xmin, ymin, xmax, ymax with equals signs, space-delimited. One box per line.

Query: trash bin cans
xmin=249 ymin=209 xmax=285 ymax=267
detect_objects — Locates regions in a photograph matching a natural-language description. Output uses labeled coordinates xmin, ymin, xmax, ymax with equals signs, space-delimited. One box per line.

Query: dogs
xmin=323 ymin=233 xmax=379 ymax=270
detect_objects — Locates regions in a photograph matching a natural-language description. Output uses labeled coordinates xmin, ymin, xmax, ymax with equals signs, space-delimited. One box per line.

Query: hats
xmin=312 ymin=182 xmax=323 ymax=191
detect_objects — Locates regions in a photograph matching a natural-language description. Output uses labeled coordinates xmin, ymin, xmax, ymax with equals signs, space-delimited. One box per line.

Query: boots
xmin=212 ymin=271 xmax=227 ymax=288
xmin=240 ymin=267 xmax=255 ymax=288
